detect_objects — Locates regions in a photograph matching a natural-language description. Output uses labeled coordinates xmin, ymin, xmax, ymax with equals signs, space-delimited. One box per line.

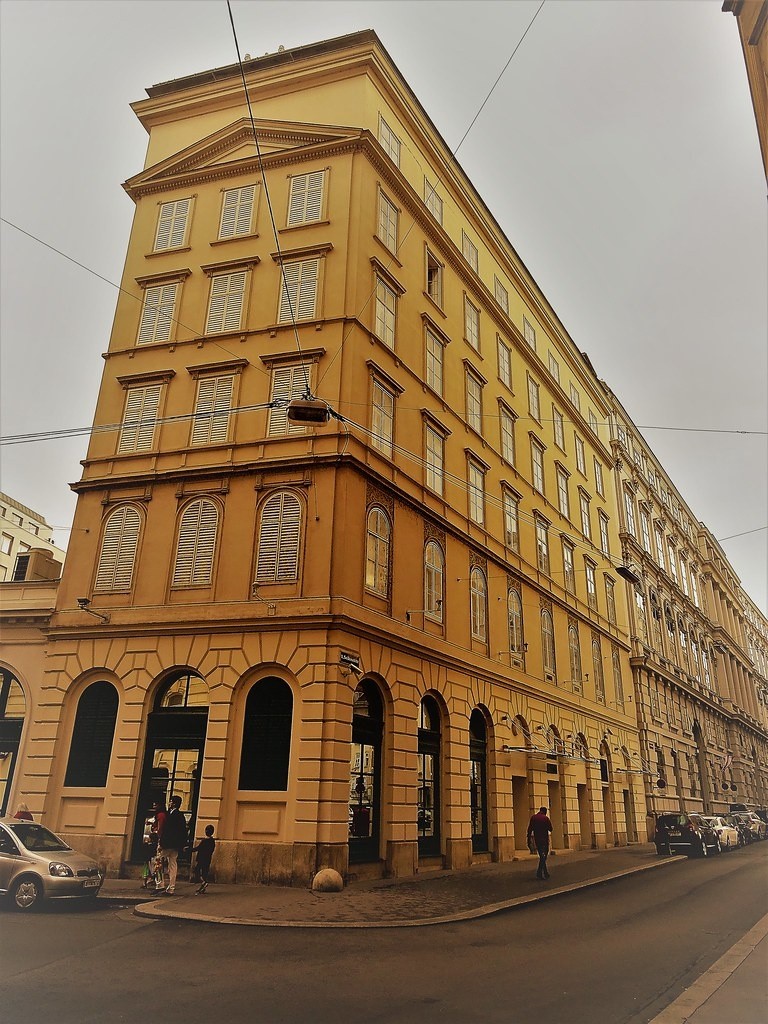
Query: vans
xmin=0 ymin=817 xmax=104 ymax=911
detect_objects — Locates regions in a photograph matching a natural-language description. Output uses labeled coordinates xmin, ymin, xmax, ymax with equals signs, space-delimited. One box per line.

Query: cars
xmin=703 ymin=816 xmax=742 ymax=852
xmin=715 ymin=813 xmax=753 ymax=846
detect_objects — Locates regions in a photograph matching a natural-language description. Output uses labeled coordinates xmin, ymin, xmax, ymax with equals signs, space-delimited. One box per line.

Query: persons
xmin=13 ymin=802 xmax=33 ymax=821
xmin=138 ymin=798 xmax=167 ymax=889
xmin=150 ymin=795 xmax=186 ymax=898
xmin=526 ymin=806 xmax=553 ymax=880
xmin=181 ymin=824 xmax=215 ymax=896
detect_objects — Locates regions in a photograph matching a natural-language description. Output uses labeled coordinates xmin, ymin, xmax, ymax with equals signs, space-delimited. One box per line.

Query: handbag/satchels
xmin=147 ymin=856 xmax=171 ymax=876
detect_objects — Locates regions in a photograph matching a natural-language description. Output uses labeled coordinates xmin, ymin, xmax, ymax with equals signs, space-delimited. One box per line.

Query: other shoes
xmin=197 ymin=882 xmax=209 ymax=894
xmin=537 ymin=875 xmax=551 ymax=881
xmin=150 ymin=889 xmax=175 ymax=897
xmin=140 ymin=884 xmax=148 ymax=889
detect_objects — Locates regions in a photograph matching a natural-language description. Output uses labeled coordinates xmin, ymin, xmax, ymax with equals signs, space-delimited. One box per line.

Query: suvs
xmin=731 ymin=811 xmax=768 ymax=841
xmin=653 ymin=811 xmax=721 ymax=859
